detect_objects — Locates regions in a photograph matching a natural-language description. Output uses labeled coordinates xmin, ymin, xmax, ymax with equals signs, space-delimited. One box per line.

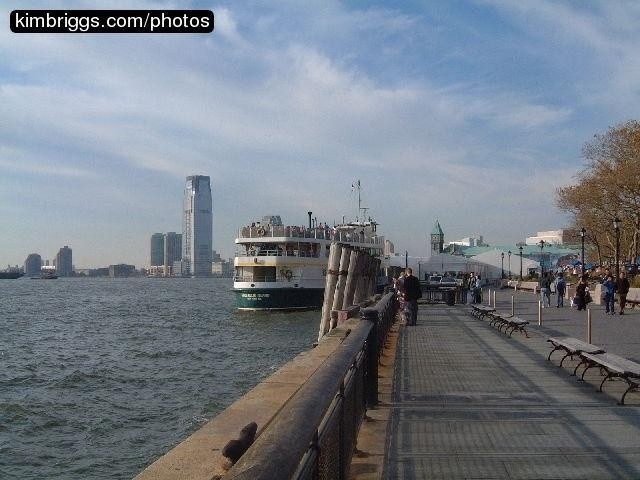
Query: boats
xmin=0 ymin=270 xmax=25 ymax=280
xmin=38 ymin=267 xmax=62 ymax=279
xmin=230 ymin=177 xmax=387 ymax=313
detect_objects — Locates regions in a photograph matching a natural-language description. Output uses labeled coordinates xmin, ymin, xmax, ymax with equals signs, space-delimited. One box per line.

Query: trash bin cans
xmin=442 ymin=289 xmax=456 ymax=306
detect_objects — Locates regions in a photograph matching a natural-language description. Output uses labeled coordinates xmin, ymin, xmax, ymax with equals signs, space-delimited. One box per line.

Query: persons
xmin=461 ymin=271 xmax=482 ymax=304
xmin=576 ymin=281 xmax=587 ymax=310
xmin=602 ymin=268 xmax=631 ymax=314
xmin=540 ymin=272 xmax=551 ymax=308
xmin=555 ymin=272 xmax=566 ymax=307
xmin=396 ymin=268 xmax=422 ymax=326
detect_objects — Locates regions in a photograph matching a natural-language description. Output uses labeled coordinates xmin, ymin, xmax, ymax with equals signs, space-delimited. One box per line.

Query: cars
xmin=427 ymin=274 xmax=464 ymax=291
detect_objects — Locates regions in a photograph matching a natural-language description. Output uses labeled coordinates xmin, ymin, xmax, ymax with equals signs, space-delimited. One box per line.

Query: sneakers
xmin=606 ymin=310 xmax=624 ymax=317
xmin=406 ymin=322 xmax=417 ymax=327
xmin=541 ymin=303 xmax=587 ymax=311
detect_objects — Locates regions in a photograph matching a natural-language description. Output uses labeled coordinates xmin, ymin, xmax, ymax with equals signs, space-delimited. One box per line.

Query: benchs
xmin=469 ymin=302 xmax=531 ymax=339
xmin=576 ymin=350 xmax=640 ymax=405
xmin=546 ymin=337 xmax=607 ymax=378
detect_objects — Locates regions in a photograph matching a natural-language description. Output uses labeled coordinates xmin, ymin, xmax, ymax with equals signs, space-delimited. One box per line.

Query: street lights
xmin=579 ymin=226 xmax=588 ymax=281
xmin=612 ymin=216 xmax=622 ymax=284
xmin=539 ymin=239 xmax=545 ymax=282
xmin=501 ymin=246 xmax=524 ymax=281
